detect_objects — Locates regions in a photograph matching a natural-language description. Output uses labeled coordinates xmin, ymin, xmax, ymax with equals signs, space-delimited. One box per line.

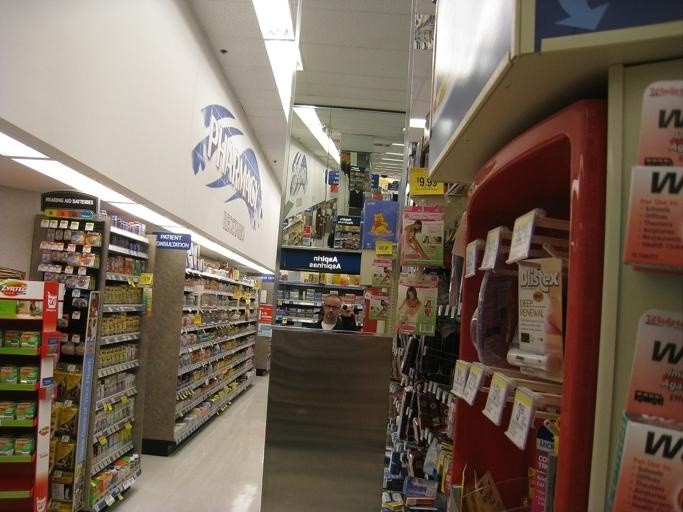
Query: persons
xmin=305 ymin=293 xmax=358 ymax=332
xmin=375 ymin=299 xmax=390 ymax=317
xmin=376 ymin=264 xmax=391 ymax=285
xmin=422 ymin=236 xmax=429 ymax=243
xmin=424 ymin=300 xmax=432 ymax=308
xmin=538 ymin=259 xmax=564 ymax=356
xmin=402 ymin=216 xmax=431 ymax=263
xmin=396 ymin=283 xmax=422 ymax=318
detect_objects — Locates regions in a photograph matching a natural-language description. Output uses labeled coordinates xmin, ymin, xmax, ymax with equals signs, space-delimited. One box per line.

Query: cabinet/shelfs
xmin=32 ymin=213 xmax=155 ymax=509
xmin=280 ymin=200 xmax=339 ymax=248
xmin=381 ymin=180 xmax=467 ymax=512
xmin=272 ymin=248 xmax=366 ymax=334
xmin=454 ymin=71 xmax=597 ymax=511
xmin=141 ymin=235 xmax=260 ymax=451
xmin=331 ymin=217 xmax=362 ymax=251
xmin=0 ymin=282 xmax=56 ymax=511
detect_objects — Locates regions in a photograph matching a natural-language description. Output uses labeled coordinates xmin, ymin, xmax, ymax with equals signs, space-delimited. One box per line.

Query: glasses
xmin=324 ymin=305 xmax=339 ymax=310
